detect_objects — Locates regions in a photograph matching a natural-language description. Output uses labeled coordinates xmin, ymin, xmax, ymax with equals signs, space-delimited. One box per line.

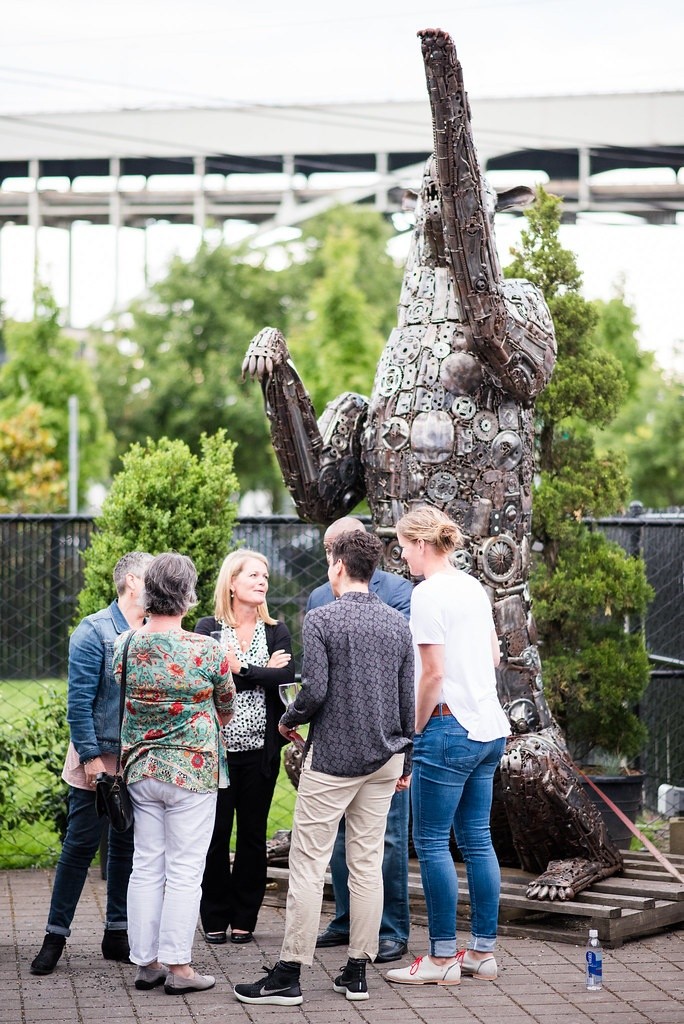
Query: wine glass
xmin=279 ymin=683 xmax=298 ymax=731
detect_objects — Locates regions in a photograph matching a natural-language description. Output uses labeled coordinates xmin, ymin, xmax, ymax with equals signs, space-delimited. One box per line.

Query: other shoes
xmin=101 ymin=929 xmax=134 ymax=964
xmin=135 ymin=964 xmax=171 ymax=990
xmin=31 ymin=934 xmax=66 ymax=973
xmin=163 ymin=971 xmax=216 ymax=994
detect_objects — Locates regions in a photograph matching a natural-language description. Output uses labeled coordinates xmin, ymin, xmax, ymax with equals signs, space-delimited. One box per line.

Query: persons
xmin=303 ymin=516 xmax=415 ymax=963
xmin=112 ymin=553 xmax=237 ymax=996
xmin=233 ymin=528 xmax=416 ymax=1005
xmin=193 ymin=549 xmax=295 ymax=946
xmin=386 ymin=507 xmax=512 ymax=987
xmin=30 ymin=549 xmax=154 ymax=978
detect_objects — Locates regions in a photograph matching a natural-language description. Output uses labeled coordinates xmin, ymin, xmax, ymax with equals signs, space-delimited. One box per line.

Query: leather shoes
xmin=375 ymin=939 xmax=407 ymax=962
xmin=456 ymin=951 xmax=497 ymax=980
xmin=205 ymin=932 xmax=227 ymax=943
xmin=316 ymin=930 xmax=349 ymax=947
xmin=231 ymin=931 xmax=253 ymax=942
xmin=385 ymin=955 xmax=461 ymax=986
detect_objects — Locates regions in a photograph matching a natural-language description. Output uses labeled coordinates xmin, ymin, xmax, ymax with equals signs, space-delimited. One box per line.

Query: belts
xmin=430 ymin=703 xmax=452 ymax=716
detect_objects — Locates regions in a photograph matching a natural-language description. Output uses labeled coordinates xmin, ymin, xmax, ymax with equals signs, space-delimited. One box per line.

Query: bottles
xmin=584 ymin=929 xmax=603 ymax=992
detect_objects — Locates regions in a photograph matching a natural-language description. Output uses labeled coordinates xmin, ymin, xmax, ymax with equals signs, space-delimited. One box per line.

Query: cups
xmin=210 ymin=630 xmax=228 ymax=652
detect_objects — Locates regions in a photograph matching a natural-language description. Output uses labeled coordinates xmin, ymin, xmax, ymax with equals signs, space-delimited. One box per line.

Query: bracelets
xmin=79 ymin=755 xmax=100 ymax=765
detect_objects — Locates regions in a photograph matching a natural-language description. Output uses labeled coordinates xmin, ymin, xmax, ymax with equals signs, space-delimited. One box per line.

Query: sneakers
xmin=234 ymin=961 xmax=303 ymax=1006
xmin=333 ymin=958 xmax=369 ymax=1000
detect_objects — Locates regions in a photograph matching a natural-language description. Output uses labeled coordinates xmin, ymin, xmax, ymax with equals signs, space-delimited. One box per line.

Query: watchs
xmin=238 ymin=662 xmax=249 ymax=677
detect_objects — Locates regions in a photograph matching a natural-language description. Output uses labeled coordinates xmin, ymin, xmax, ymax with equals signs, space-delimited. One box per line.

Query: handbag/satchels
xmin=94 ymin=772 xmax=134 ymax=832
